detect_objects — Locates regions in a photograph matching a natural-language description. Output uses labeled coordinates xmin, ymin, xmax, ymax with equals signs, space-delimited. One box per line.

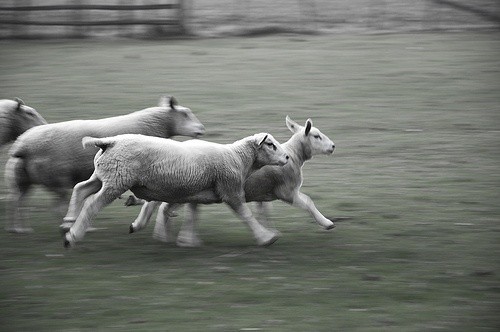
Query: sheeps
xmin=129 ymin=114 xmax=335 ymax=242
xmin=0 ymin=97 xmax=49 ymax=148
xmin=3 ymin=96 xmax=207 ymax=241
xmin=60 ymin=131 xmax=290 ymax=248
